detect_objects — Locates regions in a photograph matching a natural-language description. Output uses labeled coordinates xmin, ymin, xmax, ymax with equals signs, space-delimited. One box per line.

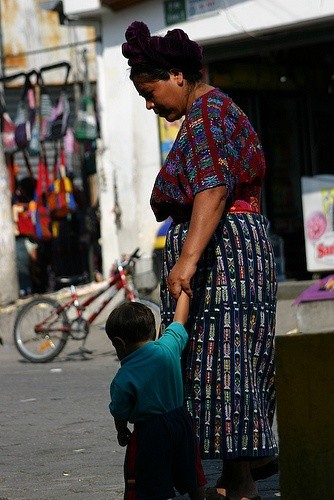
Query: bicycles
xmin=13 ymin=247 xmax=160 ymax=363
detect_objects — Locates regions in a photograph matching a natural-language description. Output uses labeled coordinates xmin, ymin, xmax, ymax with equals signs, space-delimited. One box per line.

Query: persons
xmin=122 ymin=20 xmax=280 ymax=498
xmin=10 ymin=174 xmax=97 ymax=298
xmin=106 ymin=285 xmax=208 ymax=500
